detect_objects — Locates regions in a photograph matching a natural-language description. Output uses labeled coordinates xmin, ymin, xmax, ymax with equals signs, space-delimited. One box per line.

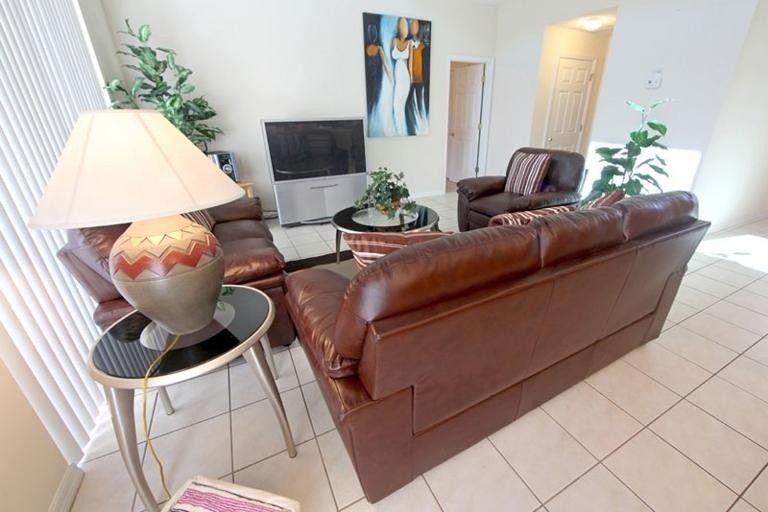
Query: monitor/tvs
xmin=260 ymin=115 xmax=367 ymax=184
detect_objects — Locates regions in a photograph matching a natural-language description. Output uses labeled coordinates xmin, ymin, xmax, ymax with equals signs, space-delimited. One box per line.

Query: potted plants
xmin=355 ymin=164 xmax=418 ymax=222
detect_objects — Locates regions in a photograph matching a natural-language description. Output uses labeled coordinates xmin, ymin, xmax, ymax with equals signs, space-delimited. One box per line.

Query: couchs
xmin=277 ymin=188 xmax=711 ymax=505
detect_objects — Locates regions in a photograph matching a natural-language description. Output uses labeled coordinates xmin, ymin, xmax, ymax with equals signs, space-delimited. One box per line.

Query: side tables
xmin=81 ymin=283 xmax=299 ymax=512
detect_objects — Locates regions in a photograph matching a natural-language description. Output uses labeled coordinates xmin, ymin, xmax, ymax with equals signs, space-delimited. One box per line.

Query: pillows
xmin=179 ymin=211 xmax=217 ymax=235
xmin=504 ymin=150 xmax=551 ymax=197
xmin=583 ymin=188 xmax=623 ymax=208
xmin=341 ymin=230 xmax=454 ymax=272
xmin=489 ymin=204 xmax=574 ymax=228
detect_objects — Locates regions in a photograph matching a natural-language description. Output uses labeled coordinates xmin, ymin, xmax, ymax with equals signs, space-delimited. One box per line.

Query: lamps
xmin=29 ymin=108 xmax=247 ymax=335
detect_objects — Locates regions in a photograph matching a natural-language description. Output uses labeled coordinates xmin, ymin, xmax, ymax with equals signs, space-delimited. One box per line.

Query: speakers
xmin=207 ymin=150 xmax=241 ymax=184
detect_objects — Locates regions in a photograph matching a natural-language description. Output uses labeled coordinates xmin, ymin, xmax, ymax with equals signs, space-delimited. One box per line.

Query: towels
xmin=162 ymin=474 xmax=298 ymax=512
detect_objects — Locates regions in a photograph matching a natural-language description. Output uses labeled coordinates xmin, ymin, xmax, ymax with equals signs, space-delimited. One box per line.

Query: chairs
xmin=57 ymin=191 xmax=291 ymax=365
xmin=454 ymin=146 xmax=588 ymax=231
